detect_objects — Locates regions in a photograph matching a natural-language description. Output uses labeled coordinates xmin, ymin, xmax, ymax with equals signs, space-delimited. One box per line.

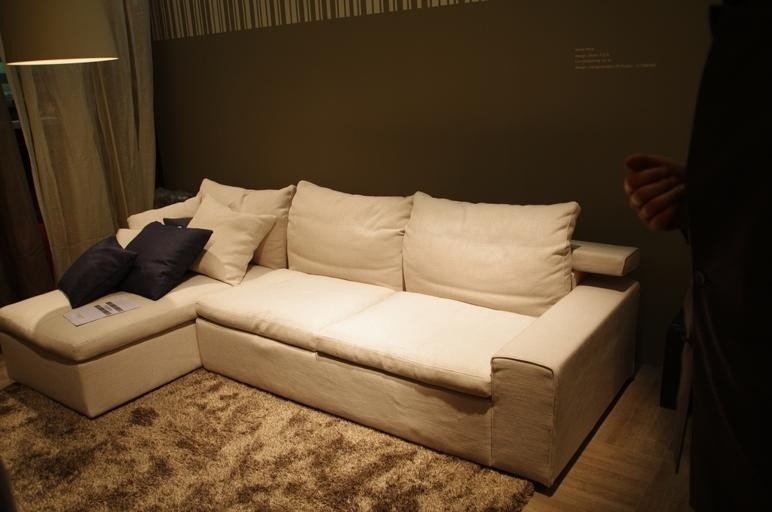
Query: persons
xmin=622 ymin=1 xmax=772 ymax=510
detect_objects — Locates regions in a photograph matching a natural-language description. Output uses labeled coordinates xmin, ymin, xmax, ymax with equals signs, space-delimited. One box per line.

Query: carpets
xmin=0 ymin=368 xmax=535 ymax=511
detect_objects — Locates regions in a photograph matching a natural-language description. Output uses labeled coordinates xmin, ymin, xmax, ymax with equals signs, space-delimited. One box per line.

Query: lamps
xmin=1 ymin=0 xmax=120 ymax=66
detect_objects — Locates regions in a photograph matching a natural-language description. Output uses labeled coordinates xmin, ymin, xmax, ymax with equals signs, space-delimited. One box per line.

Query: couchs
xmin=0 ymin=202 xmax=639 ymax=489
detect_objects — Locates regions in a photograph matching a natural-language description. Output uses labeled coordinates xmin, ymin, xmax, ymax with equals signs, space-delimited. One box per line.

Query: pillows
xmin=287 ymin=181 xmax=414 ymax=293
xmin=402 ymin=191 xmax=582 ymax=318
xmin=58 ymin=178 xmax=297 ymax=310
